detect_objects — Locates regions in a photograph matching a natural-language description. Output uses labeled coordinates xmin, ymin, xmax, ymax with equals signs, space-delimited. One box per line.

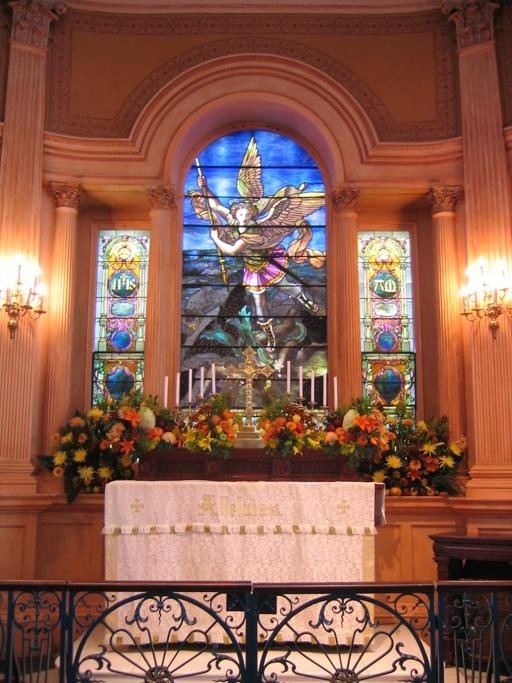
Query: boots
xmin=257 ymin=318 xmax=276 ymax=353
xmin=289 ymin=283 xmax=319 ymax=314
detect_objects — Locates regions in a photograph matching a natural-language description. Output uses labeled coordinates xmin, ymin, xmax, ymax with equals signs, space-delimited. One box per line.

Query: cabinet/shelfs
xmin=428 ymin=531 xmax=512 ymax=677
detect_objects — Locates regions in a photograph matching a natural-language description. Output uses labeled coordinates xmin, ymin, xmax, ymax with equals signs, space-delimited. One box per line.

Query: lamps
xmin=461 ymin=256 xmax=512 ymax=339
xmin=0 ymin=254 xmax=47 ymax=339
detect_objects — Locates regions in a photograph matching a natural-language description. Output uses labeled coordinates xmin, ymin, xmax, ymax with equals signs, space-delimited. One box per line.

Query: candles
xmin=287 ymin=361 xmax=338 ymax=411
xmin=164 ymin=362 xmax=215 ymax=410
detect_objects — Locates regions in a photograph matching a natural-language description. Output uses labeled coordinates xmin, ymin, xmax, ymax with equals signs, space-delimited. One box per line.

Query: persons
xmin=198 ymin=174 xmax=320 ymax=354
